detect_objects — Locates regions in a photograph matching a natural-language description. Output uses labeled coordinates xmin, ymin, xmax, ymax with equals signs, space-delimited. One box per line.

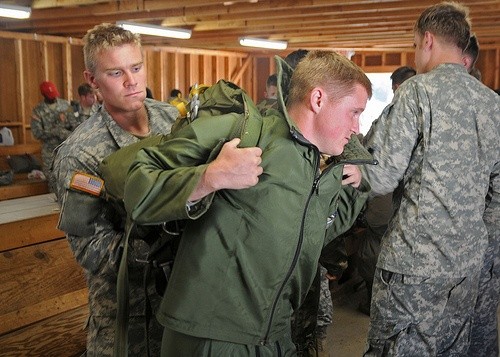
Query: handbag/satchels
xmin=7 ymin=152 xmax=41 ymax=173
xmin=356 ymin=222 xmax=388 ymax=266
xmin=0 ymin=170 xmax=13 ymax=185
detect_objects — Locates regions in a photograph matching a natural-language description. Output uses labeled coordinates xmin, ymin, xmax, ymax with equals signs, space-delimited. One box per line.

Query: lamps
xmin=116 ymin=21 xmax=192 ymax=42
xmin=0 ymin=5 xmax=32 ymax=20
xmin=240 ymin=37 xmax=288 ymax=50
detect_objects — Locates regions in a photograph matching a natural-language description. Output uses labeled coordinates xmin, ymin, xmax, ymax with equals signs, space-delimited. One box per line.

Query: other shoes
xmin=358 ymin=296 xmax=372 ymax=315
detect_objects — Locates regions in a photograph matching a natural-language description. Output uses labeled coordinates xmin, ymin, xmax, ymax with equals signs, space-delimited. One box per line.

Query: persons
xmin=50 ymin=24 xmax=379 ymax=357
xmin=347 ymin=3 xmax=500 ymax=357
xmin=30 ymin=79 xmax=101 ymax=203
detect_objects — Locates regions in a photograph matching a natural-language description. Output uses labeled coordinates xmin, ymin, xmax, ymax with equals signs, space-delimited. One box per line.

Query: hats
xmin=40 ymin=81 xmax=60 ymax=99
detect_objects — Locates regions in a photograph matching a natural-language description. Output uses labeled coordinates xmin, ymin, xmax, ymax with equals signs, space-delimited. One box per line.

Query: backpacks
xmin=98 ymin=79 xmax=264 ymax=288
